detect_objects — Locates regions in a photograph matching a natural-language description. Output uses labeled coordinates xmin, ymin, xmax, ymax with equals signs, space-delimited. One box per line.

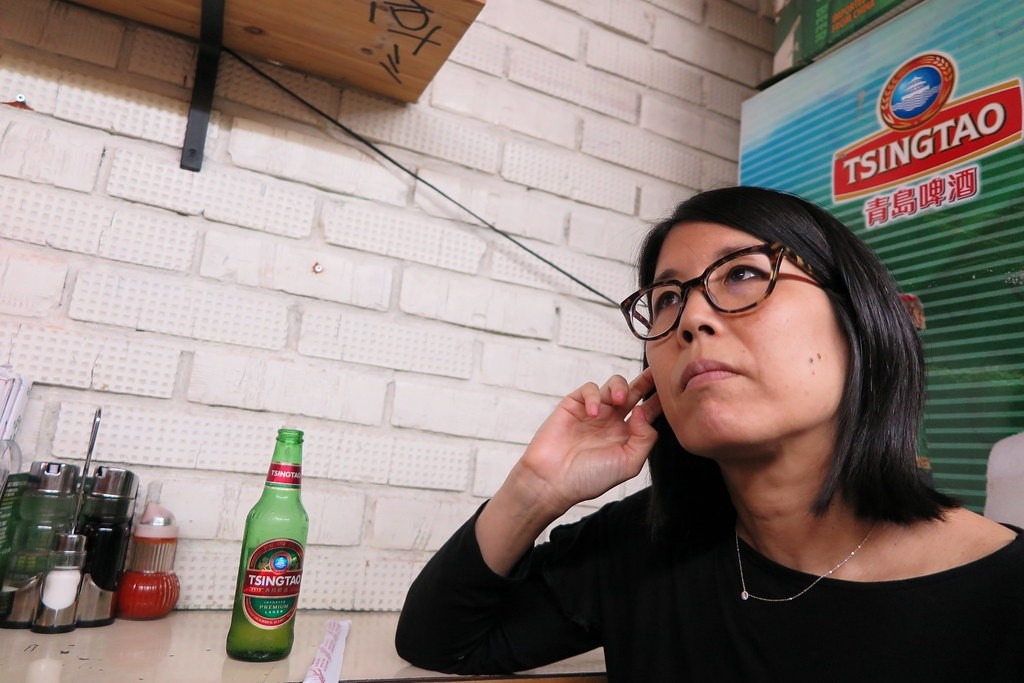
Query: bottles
xmin=31 ymin=533 xmax=87 ymax=633
xmin=0 ymin=461 xmax=80 ymax=628
xmin=72 ymin=466 xmax=134 ymax=628
xmin=226 ymin=429 xmax=309 ymax=662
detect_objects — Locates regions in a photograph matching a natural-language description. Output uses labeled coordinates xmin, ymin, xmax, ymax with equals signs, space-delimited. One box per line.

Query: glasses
xmin=620 ymin=242 xmax=832 ymax=340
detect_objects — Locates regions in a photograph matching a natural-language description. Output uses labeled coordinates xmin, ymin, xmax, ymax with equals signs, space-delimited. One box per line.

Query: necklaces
xmin=735 ymin=522 xmax=878 ymax=602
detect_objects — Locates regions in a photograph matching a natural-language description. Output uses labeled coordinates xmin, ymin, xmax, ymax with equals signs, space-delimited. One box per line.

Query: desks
xmin=0 ymin=607 xmax=607 ymax=683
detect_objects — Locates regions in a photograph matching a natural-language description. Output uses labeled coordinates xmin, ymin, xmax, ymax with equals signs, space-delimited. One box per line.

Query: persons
xmin=395 ymin=186 xmax=1024 ymax=683
